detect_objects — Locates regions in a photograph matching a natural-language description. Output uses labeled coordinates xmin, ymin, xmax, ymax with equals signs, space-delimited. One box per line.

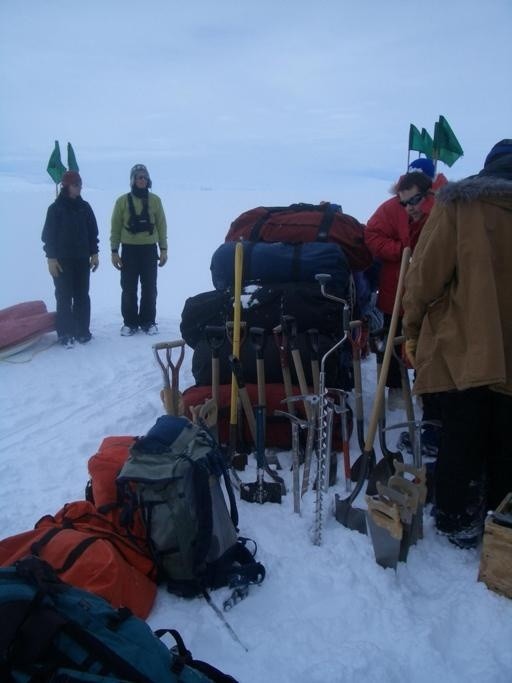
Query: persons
xmin=109 ymin=163 xmax=168 ymax=337
xmin=388 ymin=172 xmax=449 ymax=457
xmin=364 ymin=157 xmax=435 ymax=412
xmin=40 ymin=170 xmax=100 ymax=351
xmin=398 ymin=137 xmax=512 ymax=552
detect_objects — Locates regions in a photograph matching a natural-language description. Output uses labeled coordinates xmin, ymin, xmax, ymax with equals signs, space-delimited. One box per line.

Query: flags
xmin=46 ymin=143 xmax=66 ymax=186
xmin=410 ymin=114 xmax=464 ymax=167
xmin=67 ymin=141 xmax=80 ymax=172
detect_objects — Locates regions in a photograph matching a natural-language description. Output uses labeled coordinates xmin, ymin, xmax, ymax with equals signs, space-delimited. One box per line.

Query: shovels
xmin=225 ymin=313 xmax=404 ymax=504
xmin=334 ymin=246 xmax=410 ymax=535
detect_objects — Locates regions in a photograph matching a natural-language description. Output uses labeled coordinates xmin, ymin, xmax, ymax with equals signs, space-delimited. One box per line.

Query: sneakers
xmin=76 ymin=337 xmax=92 ymax=344
xmin=386 ymin=385 xmax=510 ymax=548
xmin=139 ymin=325 xmax=158 ymax=336
xmin=121 ymin=326 xmax=138 ymax=336
xmin=58 ymin=336 xmax=74 ymax=349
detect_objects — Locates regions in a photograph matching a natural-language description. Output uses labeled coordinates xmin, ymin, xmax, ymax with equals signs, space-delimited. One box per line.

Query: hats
xmin=130 ymin=165 xmax=152 ymax=188
xmin=484 ymin=139 xmax=512 ymax=168
xmin=408 ymin=158 xmax=435 ymax=179
xmin=62 ymin=172 xmax=82 ymax=188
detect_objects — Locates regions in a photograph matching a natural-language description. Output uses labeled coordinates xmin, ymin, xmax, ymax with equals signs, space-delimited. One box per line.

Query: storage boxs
xmin=476 ymin=492 xmax=512 ymax=600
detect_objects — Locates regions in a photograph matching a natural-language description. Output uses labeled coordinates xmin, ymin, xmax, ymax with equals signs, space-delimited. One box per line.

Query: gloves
xmin=159 ymin=250 xmax=167 ymax=266
xmin=90 ymin=254 xmax=99 ymax=272
xmin=405 ymin=339 xmax=417 ymax=371
xmin=48 ymin=258 xmax=63 ymax=277
xmin=111 ymin=252 xmax=123 ymax=270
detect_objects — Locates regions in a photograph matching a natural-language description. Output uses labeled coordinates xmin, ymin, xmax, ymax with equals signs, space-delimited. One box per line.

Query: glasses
xmin=136 ymin=174 xmax=149 ymax=180
xmin=399 ymin=193 xmax=435 ymax=208
